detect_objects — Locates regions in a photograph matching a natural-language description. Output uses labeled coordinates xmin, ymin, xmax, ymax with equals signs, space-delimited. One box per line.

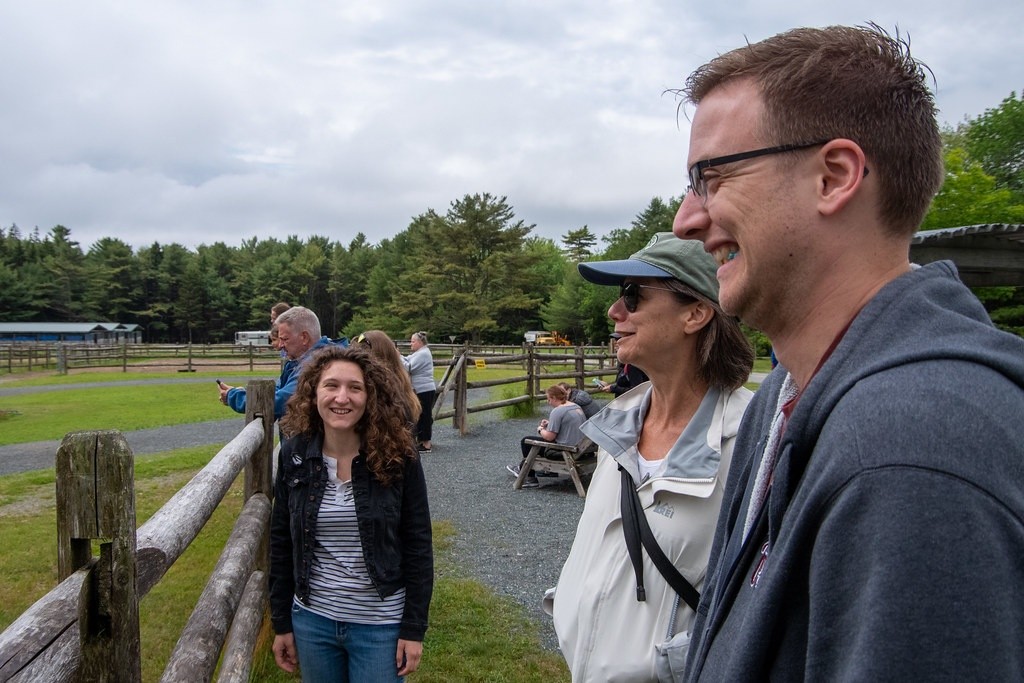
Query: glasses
xmin=358 ymin=332 xmax=372 ymax=349
xmin=619 ymin=282 xmax=675 ymax=313
xmin=683 ymin=139 xmax=870 ymax=206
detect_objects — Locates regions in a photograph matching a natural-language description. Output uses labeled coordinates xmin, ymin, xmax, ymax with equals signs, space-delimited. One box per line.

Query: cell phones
xmin=215 ymin=378 xmax=223 ymax=385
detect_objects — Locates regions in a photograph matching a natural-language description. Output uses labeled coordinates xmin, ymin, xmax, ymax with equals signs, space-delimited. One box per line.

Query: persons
xmin=274 ymin=346 xmax=436 ymax=683
xmin=596 ymin=332 xmax=649 ymax=398
xmin=537 ymin=382 xmax=600 ymax=478
xmin=661 ymin=19 xmax=1023 ymax=682
xmin=543 ymin=230 xmax=756 ymax=683
xmin=506 ymin=385 xmax=587 ymax=488
xmin=216 ymin=302 xmax=436 ymax=454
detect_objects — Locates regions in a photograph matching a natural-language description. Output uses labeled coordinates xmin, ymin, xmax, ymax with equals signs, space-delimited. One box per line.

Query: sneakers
xmin=506 ymin=463 xmax=521 ymax=478
xmin=522 ymin=476 xmax=539 ymax=487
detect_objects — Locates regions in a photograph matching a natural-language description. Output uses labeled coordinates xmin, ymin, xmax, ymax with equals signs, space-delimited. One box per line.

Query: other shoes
xmin=418 ymin=442 xmax=432 ymax=453
xmin=536 ymin=469 xmax=558 ymax=477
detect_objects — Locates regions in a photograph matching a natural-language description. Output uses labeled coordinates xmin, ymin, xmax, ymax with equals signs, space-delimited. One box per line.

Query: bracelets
xmin=538 ymin=428 xmax=545 ymax=435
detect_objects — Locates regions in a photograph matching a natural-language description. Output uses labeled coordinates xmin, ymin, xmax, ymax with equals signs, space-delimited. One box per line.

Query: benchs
xmin=513 ymin=435 xmax=598 ymax=498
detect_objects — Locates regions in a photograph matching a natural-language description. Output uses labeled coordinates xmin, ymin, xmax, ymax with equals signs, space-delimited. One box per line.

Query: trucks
xmin=523 ymin=331 xmax=558 ymax=345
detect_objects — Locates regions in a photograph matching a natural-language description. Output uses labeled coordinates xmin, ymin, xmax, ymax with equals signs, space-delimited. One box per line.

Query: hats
xmin=578 ymin=231 xmax=720 ymax=304
xmin=609 ymin=332 xmax=622 ymax=340
